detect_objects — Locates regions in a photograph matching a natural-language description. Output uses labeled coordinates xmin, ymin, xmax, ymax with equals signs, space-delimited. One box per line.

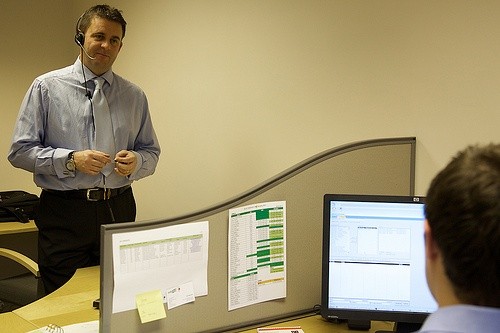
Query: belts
xmin=42 ymin=184 xmax=131 ymax=202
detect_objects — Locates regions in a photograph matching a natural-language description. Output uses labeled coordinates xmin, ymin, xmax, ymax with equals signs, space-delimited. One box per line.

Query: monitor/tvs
xmin=321 ymin=193 xmax=439 ymax=323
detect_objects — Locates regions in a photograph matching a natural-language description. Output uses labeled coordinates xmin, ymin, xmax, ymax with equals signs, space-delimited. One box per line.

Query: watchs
xmin=65 ymin=152 xmax=77 ymax=173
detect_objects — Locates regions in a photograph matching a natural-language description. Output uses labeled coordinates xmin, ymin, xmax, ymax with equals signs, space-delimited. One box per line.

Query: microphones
xmin=76 ymin=39 xmax=96 ymax=59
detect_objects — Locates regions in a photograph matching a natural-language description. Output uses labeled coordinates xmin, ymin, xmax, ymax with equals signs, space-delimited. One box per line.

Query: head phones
xmin=75 ymin=12 xmax=85 ymax=44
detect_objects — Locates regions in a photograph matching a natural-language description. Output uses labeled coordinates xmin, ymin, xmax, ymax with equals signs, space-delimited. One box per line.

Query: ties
xmin=90 ymin=77 xmax=115 ymax=177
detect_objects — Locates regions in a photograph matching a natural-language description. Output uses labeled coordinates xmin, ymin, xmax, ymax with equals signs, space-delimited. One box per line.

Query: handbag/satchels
xmin=0 ymin=191 xmax=39 ymax=223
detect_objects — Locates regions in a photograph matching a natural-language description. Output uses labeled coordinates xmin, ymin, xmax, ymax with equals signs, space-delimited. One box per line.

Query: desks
xmin=0 ymin=219 xmax=395 ymax=333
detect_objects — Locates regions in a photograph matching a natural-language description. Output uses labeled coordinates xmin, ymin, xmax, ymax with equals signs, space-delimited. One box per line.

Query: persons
xmin=414 ymin=142 xmax=500 ymax=333
xmin=7 ymin=4 xmax=161 ymax=296
